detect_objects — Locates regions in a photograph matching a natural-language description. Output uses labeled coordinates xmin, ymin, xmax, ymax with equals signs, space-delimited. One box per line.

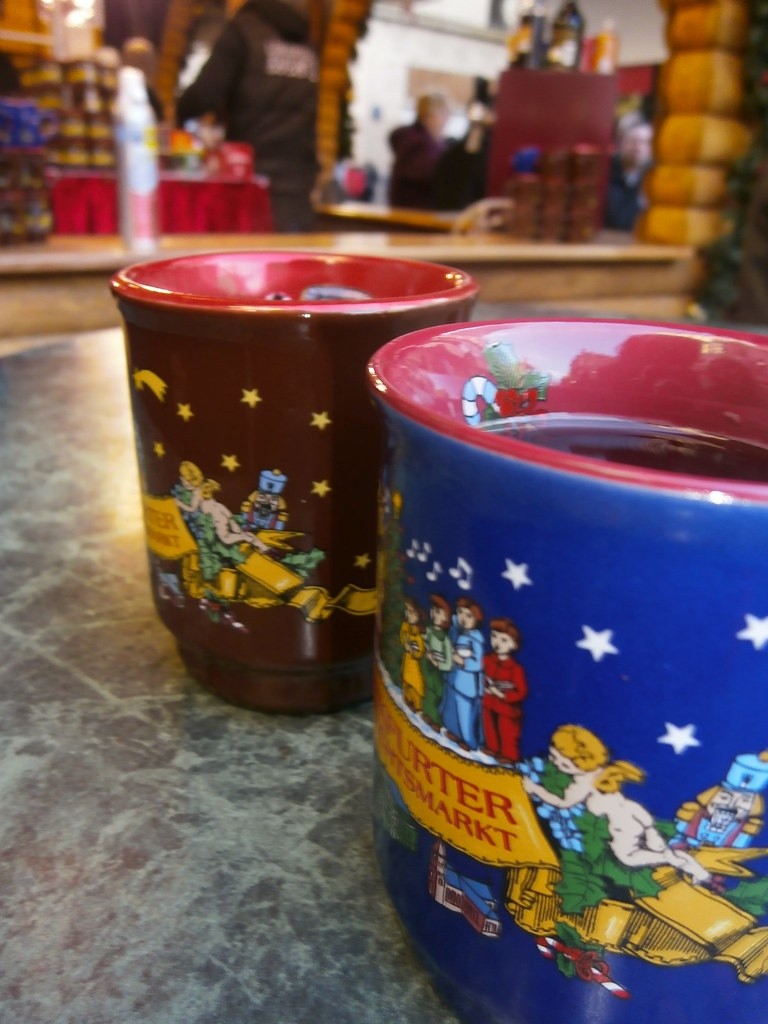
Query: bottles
xmin=594 ymin=18 xmax=618 ymax=75
xmin=542 ymin=1 xmax=585 ymax=71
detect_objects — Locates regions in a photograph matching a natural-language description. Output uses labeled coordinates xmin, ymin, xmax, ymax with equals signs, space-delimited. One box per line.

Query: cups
xmin=110 ymin=251 xmax=482 ymax=714
xmin=369 ymin=316 xmax=767 ymax=1023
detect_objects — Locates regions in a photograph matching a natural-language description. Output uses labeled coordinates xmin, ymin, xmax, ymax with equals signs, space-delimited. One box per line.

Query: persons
xmin=604 ymin=111 xmax=652 ymax=228
xmin=175 ymin=1 xmax=316 ymax=229
xmin=389 ymin=95 xmax=452 ymax=212
